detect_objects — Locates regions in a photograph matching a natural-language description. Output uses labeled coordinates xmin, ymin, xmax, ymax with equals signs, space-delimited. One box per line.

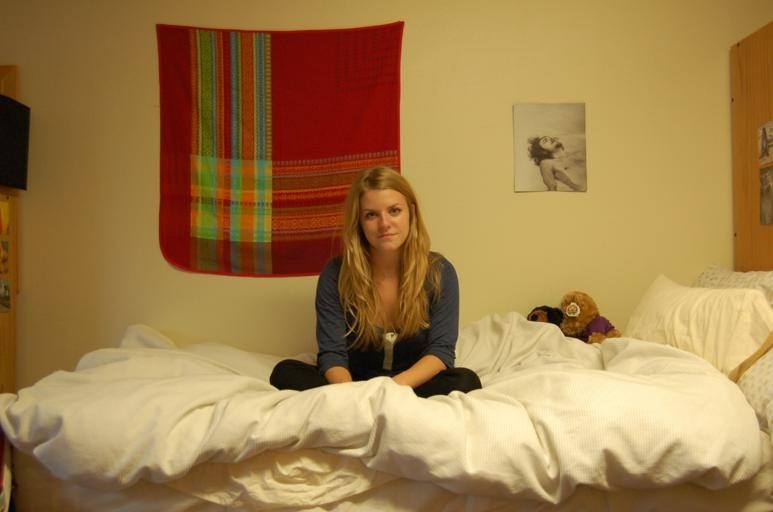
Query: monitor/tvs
xmin=0 ymin=95 xmax=31 ymax=191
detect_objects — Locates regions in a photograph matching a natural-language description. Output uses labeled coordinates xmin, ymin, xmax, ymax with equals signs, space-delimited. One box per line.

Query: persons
xmin=268 ymin=165 xmax=481 ymax=399
xmin=526 ymin=135 xmax=586 ymax=192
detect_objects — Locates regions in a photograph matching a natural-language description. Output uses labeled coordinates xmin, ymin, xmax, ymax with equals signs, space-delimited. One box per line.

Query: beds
xmin=0 ymin=323 xmax=772 ymax=512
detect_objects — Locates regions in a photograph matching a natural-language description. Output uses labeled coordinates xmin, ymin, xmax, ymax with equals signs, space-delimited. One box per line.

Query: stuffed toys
xmin=526 ymin=305 xmax=562 ymax=326
xmin=559 ymin=291 xmax=620 ymax=346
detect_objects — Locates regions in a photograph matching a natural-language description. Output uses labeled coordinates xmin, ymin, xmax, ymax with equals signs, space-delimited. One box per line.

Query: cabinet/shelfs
xmin=0 ymin=63 xmax=19 ymax=392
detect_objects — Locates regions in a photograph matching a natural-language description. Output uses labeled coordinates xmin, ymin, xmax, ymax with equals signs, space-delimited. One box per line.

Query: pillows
xmin=735 ymin=346 xmax=773 ymax=435
xmin=690 ymin=265 xmax=773 ymax=307
xmin=615 ymin=275 xmax=773 ymax=380
